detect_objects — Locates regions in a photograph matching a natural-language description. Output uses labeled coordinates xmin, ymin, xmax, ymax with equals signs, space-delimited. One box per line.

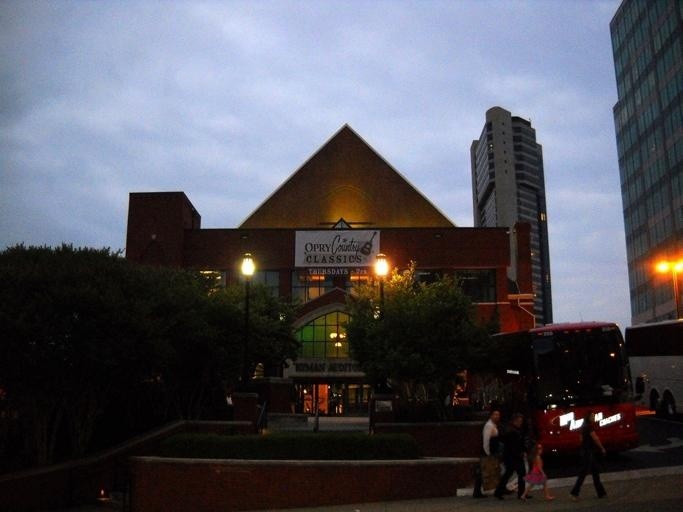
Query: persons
xmin=521 ymin=442 xmax=556 ymax=500
xmin=473 ymin=409 xmax=513 ymax=497
xmin=570 ymin=412 xmax=607 ymax=501
xmin=494 ymin=413 xmax=533 ymax=500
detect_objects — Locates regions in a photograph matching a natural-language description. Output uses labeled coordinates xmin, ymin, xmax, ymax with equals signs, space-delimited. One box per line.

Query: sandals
xmin=519 ymin=494 xmax=583 ymax=502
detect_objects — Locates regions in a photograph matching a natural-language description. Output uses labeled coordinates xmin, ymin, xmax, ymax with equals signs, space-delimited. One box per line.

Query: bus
xmin=460 ymin=316 xmax=638 ymax=462
xmin=622 ymin=320 xmax=682 ymax=430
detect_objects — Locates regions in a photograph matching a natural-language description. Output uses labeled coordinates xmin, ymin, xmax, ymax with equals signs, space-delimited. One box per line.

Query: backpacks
xmin=489 ymin=424 xmax=523 ymax=459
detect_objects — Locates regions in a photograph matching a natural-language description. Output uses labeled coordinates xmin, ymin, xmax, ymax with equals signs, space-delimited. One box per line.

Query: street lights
xmin=372 ymin=252 xmax=389 ymax=316
xmin=650 ymin=258 xmax=682 ymax=318
xmin=241 ymin=251 xmax=257 ymax=389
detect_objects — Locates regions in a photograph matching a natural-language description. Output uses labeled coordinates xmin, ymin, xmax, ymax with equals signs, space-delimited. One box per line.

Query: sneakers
xmin=494 ymin=492 xmax=505 ymax=500
xmin=473 ymin=492 xmax=489 ymax=498
